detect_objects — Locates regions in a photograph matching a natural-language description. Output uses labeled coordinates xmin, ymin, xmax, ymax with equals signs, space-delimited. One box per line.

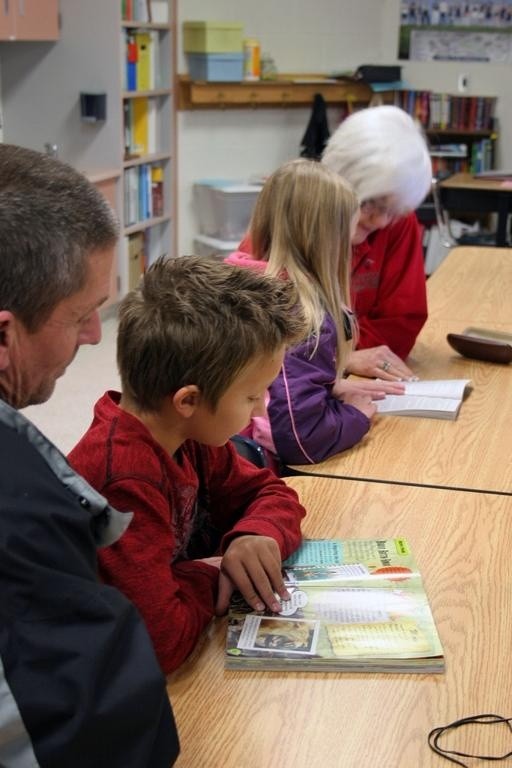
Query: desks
xmin=169 ymin=475 xmax=512 ymax=768
xmin=287 ymin=246 xmax=512 ymax=494
xmin=437 ymin=170 xmax=512 ymax=248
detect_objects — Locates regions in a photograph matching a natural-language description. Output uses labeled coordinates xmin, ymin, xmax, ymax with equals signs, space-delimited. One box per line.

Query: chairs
xmin=430 ymin=177 xmax=497 ymax=246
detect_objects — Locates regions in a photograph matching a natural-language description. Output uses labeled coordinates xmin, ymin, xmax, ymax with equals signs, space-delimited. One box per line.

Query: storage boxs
xmin=190 ymin=234 xmax=239 ymax=259
xmin=188 ymin=50 xmax=241 ymax=81
xmin=195 ymin=178 xmax=270 ymax=241
xmin=182 ymin=17 xmax=239 ymax=51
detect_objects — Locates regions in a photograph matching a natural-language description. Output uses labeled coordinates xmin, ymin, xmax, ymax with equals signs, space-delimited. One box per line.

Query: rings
xmin=381 ymin=360 xmax=391 ymax=372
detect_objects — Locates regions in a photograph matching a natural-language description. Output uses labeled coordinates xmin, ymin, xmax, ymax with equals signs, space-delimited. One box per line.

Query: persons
xmin=0 ymin=140 xmax=180 ymax=767
xmin=220 ymin=158 xmax=407 ymax=467
xmin=319 ymin=105 xmax=434 ymax=382
xmin=402 ymin=0 xmax=512 ymax=29
xmin=63 ymin=253 xmax=308 ymax=677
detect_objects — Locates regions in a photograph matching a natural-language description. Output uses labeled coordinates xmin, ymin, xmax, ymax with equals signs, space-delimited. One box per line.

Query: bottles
xmin=243 ymin=41 xmax=262 ymax=83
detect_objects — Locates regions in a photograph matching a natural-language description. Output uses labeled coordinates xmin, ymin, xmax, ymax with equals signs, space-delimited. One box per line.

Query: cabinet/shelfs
xmin=1 ymin=0 xmax=60 ymax=42
xmin=370 ymin=88 xmax=499 ymax=233
xmin=177 ymin=79 xmax=368 ymax=113
xmin=69 ymin=168 xmax=123 ymax=313
xmin=1 ymin=0 xmax=177 ymax=305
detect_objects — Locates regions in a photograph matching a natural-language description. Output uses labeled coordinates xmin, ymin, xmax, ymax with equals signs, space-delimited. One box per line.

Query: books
xmin=119 ymin=0 xmax=171 ymax=294
xmin=374 ymin=376 xmax=473 ymax=422
xmin=394 ymin=89 xmax=497 ymax=180
xmin=223 ymin=538 xmax=447 ymax=676
